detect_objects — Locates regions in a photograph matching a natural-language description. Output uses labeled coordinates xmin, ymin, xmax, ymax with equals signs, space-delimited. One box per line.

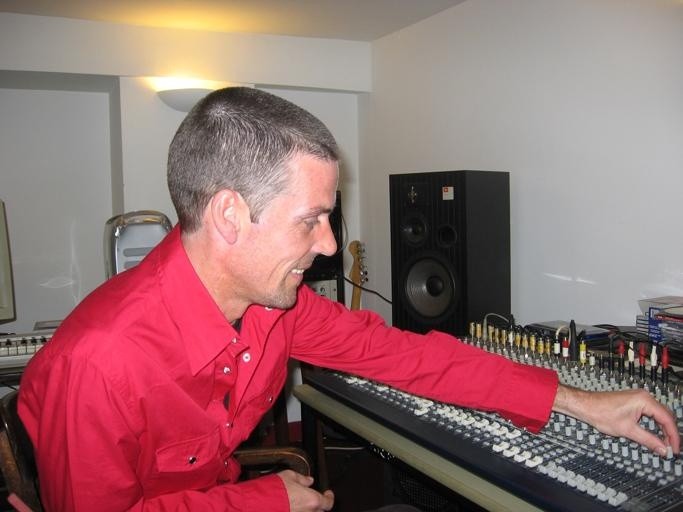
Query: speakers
xmin=390 ymin=169 xmax=510 ymax=338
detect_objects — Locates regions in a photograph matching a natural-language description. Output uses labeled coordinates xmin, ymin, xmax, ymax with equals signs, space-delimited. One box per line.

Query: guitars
xmin=348 ymin=241 xmax=368 ymax=308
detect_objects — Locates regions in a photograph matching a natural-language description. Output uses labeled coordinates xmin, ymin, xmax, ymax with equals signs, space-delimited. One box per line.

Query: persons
xmin=16 ymin=87 xmax=681 ymax=512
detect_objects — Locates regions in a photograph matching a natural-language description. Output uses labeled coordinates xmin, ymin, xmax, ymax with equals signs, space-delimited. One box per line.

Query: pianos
xmin=0 ymin=328 xmax=57 ymax=370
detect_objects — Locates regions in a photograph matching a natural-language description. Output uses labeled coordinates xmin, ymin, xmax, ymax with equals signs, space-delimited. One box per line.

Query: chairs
xmin=1 ymin=381 xmax=315 ymax=510
